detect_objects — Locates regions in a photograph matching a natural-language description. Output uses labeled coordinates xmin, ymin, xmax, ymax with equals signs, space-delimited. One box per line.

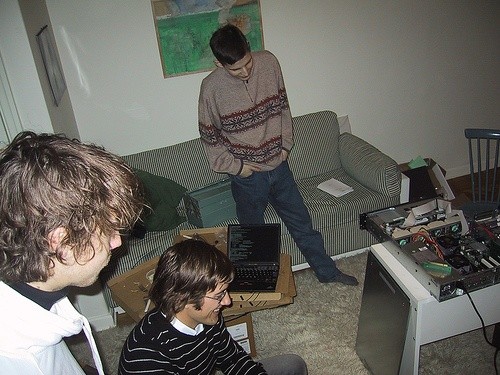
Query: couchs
xmin=98 ymin=110 xmax=402 ymax=327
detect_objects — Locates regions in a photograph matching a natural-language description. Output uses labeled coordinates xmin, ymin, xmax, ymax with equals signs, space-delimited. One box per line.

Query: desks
xmin=369 ymin=244 xmax=500 ymax=375
xmin=105 ymin=233 xmax=297 ymax=358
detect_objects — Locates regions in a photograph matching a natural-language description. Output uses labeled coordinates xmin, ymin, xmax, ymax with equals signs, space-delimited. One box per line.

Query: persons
xmin=119 ymin=239 xmax=308 ymax=375
xmin=198 ymin=25 xmax=357 ymax=287
xmin=0 ymin=131 xmax=143 ymax=375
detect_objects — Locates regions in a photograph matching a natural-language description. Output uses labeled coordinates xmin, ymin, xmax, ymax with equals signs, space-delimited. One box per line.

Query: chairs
xmin=458 ymin=129 xmax=500 ymax=220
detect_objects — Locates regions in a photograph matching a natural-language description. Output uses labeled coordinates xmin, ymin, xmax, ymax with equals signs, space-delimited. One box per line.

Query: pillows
xmin=129 ymin=168 xmax=188 ymax=232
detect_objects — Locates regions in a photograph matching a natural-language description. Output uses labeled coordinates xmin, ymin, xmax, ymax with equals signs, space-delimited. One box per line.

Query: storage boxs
xmin=184 ymin=177 xmax=237 ymax=229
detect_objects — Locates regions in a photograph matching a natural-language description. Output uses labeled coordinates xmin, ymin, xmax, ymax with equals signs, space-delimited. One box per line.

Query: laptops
xmin=223 ymin=223 xmax=282 ymax=292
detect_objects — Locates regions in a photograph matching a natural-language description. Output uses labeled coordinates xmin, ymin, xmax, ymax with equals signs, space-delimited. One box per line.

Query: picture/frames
xmin=150 ymin=0 xmax=265 ymax=79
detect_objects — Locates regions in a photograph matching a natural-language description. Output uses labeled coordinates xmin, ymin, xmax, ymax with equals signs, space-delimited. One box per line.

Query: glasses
xmin=203 ymin=289 xmax=227 ymax=301
